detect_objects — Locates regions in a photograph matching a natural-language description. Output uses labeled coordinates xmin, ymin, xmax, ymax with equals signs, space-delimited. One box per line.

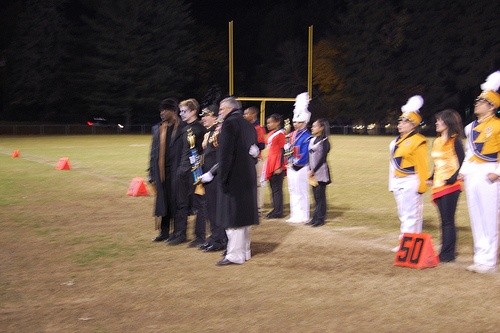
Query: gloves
xmin=198 ymin=170 xmax=215 ymax=184
xmin=249 ymin=143 xmax=260 ymax=160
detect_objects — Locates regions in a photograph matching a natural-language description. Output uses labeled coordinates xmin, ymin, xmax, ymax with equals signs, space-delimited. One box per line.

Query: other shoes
xmin=168 ymin=237 xmax=185 ymax=246
xmin=214 ymin=249 xmax=234 ymax=265
xmin=152 ymin=233 xmax=176 ymax=243
xmin=466 ymin=262 xmax=496 ymax=273
xmin=188 ymin=237 xmax=203 ymax=247
xmin=307 ymin=218 xmax=324 ymax=227
xmin=266 ymin=212 xmax=282 ymax=218
xmin=200 ymin=241 xmax=226 ymax=251
xmin=285 ymin=216 xmax=309 ymax=223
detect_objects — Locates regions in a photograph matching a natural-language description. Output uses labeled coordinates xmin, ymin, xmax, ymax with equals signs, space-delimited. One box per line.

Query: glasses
xmin=177 ymin=108 xmax=189 ymax=114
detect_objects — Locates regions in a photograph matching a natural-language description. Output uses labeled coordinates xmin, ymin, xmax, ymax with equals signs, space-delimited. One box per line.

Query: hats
xmin=292 ymin=92 xmax=312 ymax=124
xmin=159 ymin=98 xmax=178 ymax=111
xmin=201 ymin=105 xmax=218 ymax=117
xmin=474 ymin=70 xmax=500 ymax=110
xmin=397 ymin=93 xmax=424 ymax=127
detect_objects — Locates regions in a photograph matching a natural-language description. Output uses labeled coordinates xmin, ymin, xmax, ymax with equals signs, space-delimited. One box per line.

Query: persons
xmin=260 ymin=114 xmax=288 ymax=219
xmin=304 ymin=119 xmax=332 ymax=228
xmin=197 ymin=98 xmax=259 ymax=267
xmin=457 ymin=72 xmax=500 ymax=274
xmin=167 ymin=99 xmax=207 ymax=248
xmin=388 ymin=95 xmax=430 ymax=253
xmin=426 ymin=108 xmax=465 ymax=262
xmin=286 ymin=92 xmax=313 ymax=223
xmin=242 ymin=108 xmax=265 ymax=217
xmin=146 ymin=99 xmax=187 ymax=242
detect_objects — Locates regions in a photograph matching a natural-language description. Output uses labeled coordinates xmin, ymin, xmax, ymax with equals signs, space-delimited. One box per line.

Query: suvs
xmin=87 ymin=117 xmax=126 ymax=133
